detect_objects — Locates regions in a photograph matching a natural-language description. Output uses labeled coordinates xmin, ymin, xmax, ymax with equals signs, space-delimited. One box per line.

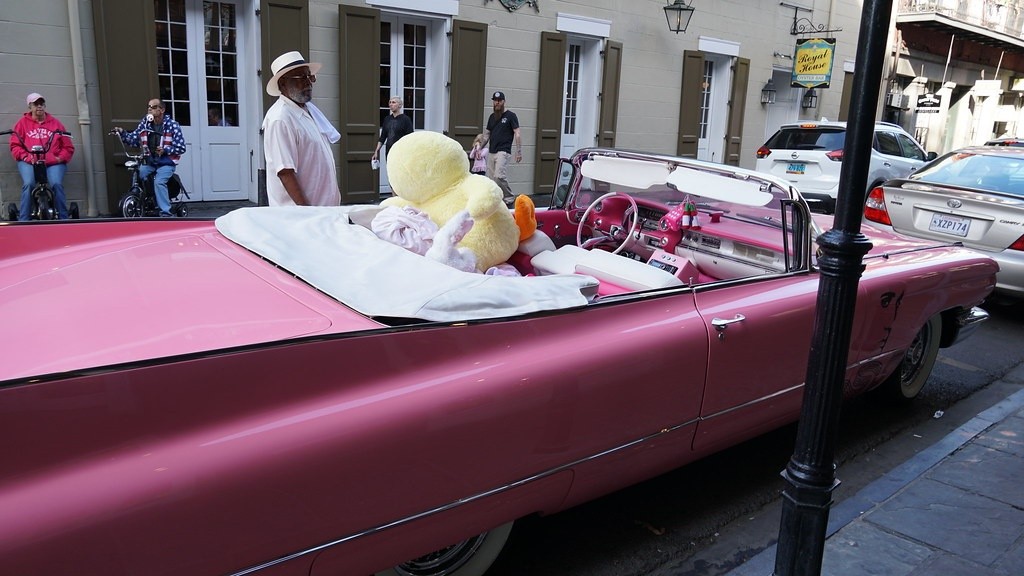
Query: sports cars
xmin=0 ymin=146 xmax=1004 ymax=576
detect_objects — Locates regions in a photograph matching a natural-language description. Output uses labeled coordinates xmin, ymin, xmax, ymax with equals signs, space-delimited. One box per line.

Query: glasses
xmin=146 ymin=104 xmax=162 ymax=109
xmin=287 ymin=75 xmax=317 ymax=83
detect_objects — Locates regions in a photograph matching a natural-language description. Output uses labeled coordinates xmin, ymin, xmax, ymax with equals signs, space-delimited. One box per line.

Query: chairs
xmin=574 ymin=247 xmax=684 ymax=298
xmin=508 ymin=227 xmax=556 ymax=277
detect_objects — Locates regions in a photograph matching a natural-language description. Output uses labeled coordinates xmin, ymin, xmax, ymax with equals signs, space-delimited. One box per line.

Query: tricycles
xmin=107 ymin=127 xmax=191 ymax=219
xmin=0 ymin=129 xmax=81 ymax=221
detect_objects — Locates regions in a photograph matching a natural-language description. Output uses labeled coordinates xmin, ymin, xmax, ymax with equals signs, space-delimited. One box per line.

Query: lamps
xmin=663 ymin=0 xmax=696 ymax=34
xmin=802 ymin=88 xmax=818 ymax=108
xmin=761 ymin=79 xmax=776 ymax=105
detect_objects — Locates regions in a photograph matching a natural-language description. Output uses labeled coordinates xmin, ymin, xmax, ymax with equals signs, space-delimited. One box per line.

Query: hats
xmin=491 ymin=91 xmax=505 ymax=100
xmin=265 ymin=50 xmax=322 ymax=97
xmin=27 ymin=93 xmax=45 ymax=107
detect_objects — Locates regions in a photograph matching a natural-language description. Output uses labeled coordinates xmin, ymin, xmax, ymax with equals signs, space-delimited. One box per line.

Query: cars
xmin=955 ymin=138 xmax=1024 ymax=185
xmin=864 ymin=147 xmax=1024 ymax=299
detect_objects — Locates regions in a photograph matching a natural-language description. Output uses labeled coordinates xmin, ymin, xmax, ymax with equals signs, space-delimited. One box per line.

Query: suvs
xmin=754 ymin=121 xmax=941 ymax=213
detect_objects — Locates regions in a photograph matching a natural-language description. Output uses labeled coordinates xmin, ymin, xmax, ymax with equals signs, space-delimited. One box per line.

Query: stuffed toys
xmin=386 ymin=130 xmax=536 ymax=273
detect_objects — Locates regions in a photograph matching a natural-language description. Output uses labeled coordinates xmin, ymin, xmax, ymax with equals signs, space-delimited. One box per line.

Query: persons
xmin=473 ymin=91 xmax=522 ymax=206
xmin=209 ymin=107 xmax=233 ymax=126
xmin=9 ymin=92 xmax=75 ymax=221
xmin=470 ymin=134 xmax=489 ymax=176
xmin=372 ymin=96 xmax=414 ymax=197
xmin=113 ymin=98 xmax=186 ymax=217
xmin=263 ymin=51 xmax=342 ymax=206
